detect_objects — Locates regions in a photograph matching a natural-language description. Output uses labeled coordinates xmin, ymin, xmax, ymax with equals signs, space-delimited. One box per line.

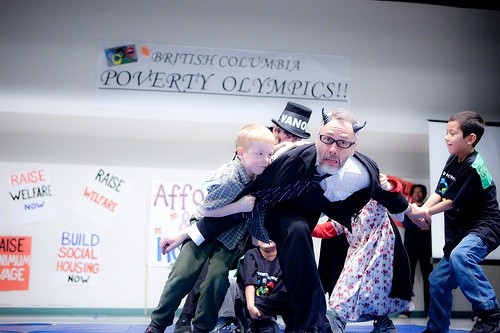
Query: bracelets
xmin=424 ymin=209 xmax=431 ymax=219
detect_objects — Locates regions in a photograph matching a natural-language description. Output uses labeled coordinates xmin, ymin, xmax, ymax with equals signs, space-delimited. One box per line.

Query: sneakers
xmin=369 ymin=319 xmax=397 ymax=333
xmin=470 ymin=313 xmax=500 ymax=333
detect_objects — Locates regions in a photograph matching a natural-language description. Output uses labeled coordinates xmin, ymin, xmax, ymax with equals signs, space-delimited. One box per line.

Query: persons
xmin=143 ymin=102 xmax=432 ymax=333
xmin=411 ymin=111 xmax=500 ymax=333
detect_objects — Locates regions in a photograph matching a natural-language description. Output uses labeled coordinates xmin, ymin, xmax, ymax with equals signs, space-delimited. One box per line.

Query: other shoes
xmin=144 ymin=321 xmax=163 ymax=333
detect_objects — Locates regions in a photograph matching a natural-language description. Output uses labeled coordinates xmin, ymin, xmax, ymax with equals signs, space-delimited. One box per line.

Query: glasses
xmin=318 ymin=133 xmax=359 ymax=151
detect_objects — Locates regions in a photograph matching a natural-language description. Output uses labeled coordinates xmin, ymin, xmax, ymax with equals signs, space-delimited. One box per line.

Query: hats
xmin=270 ymin=101 xmax=312 ymax=139
